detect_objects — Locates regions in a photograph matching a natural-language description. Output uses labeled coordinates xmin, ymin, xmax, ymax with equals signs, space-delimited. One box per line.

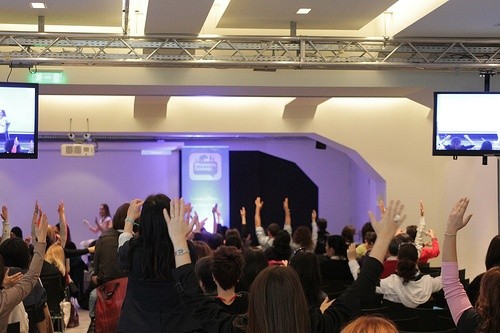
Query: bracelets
xmin=444 ymin=233 xmax=456 ymax=236
xmin=125 ymin=217 xmax=139 ymax=225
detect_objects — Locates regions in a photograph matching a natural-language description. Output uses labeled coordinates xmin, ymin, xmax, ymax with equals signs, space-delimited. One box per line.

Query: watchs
xmin=174 ymin=247 xmax=189 ymax=257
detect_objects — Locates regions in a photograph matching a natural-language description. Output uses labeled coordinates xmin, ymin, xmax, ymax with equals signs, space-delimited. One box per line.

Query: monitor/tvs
xmin=432 ymin=92 xmax=500 ymax=157
xmin=0 ymin=82 xmax=39 ymax=160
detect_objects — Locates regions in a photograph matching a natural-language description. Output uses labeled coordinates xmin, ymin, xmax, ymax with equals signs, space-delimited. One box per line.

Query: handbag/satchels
xmin=60 ymin=299 xmax=71 ymax=327
xmin=94 ymin=278 xmax=128 ymax=333
xmin=67 ymin=297 xmax=79 ymax=328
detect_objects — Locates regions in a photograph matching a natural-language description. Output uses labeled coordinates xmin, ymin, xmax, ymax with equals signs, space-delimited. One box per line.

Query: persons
xmin=0 ymin=109 xmax=35 ymax=153
xmin=0 ymin=194 xmax=500 ymax=333
xmin=440 ymin=134 xmax=492 ymax=150
